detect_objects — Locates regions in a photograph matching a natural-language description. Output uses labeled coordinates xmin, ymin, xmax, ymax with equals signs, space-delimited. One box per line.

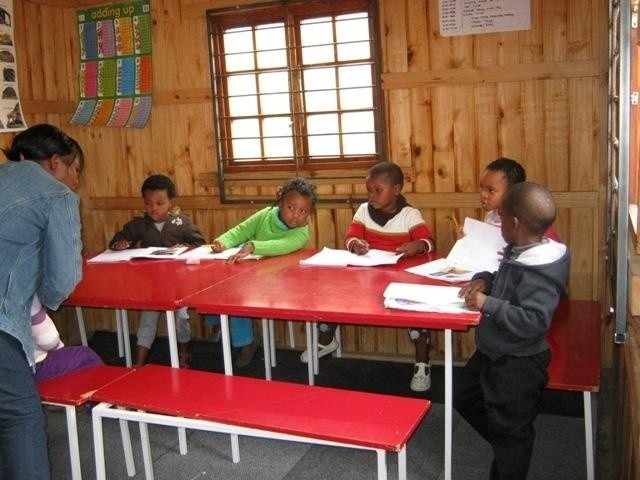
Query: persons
xmin=203 ymin=176 xmax=320 ymax=372
xmin=480 ymin=157 xmax=565 ymax=244
xmin=0 ymin=123 xmax=86 ymax=480
xmin=29 ymin=289 xmax=105 ymax=383
xmin=301 ymin=161 xmax=439 ymax=393
xmin=109 ymin=174 xmax=210 ymax=370
xmin=454 ymin=180 xmax=571 ymax=480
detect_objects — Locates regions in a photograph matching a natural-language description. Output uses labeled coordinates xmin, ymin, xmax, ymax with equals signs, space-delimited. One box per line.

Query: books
xmin=300 ymin=245 xmax=407 ymax=267
xmin=405 ymin=217 xmax=510 ymax=286
xmin=384 ymin=281 xmax=482 ymax=315
xmin=173 ymin=245 xmax=264 ymax=262
xmin=86 ymin=245 xmax=189 ymax=266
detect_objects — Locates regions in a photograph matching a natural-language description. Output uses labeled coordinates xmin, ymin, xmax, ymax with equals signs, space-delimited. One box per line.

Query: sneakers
xmin=236 ymin=342 xmax=257 ymax=367
xmin=300 ymin=336 xmax=339 ymax=364
xmin=179 ymin=352 xmax=192 ymax=369
xmin=410 ymin=361 xmax=432 ymax=392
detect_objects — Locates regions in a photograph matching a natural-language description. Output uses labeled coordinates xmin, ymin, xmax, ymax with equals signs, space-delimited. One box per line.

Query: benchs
xmin=39 ymin=366 xmax=136 ymax=479
xmin=532 ymin=301 xmax=604 ymax=476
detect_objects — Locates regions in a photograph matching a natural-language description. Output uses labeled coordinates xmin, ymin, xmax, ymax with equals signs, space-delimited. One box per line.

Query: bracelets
xmin=347 ymin=237 xmax=361 ymax=252
xmin=419 ymin=238 xmax=432 ymax=254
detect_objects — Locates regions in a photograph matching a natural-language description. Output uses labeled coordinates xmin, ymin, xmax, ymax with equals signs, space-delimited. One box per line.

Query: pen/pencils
xmin=203 ymin=244 xmax=216 ymax=247
xmin=127 ymin=241 xmax=132 ymax=244
xmin=449 ymin=211 xmax=460 ymax=231
